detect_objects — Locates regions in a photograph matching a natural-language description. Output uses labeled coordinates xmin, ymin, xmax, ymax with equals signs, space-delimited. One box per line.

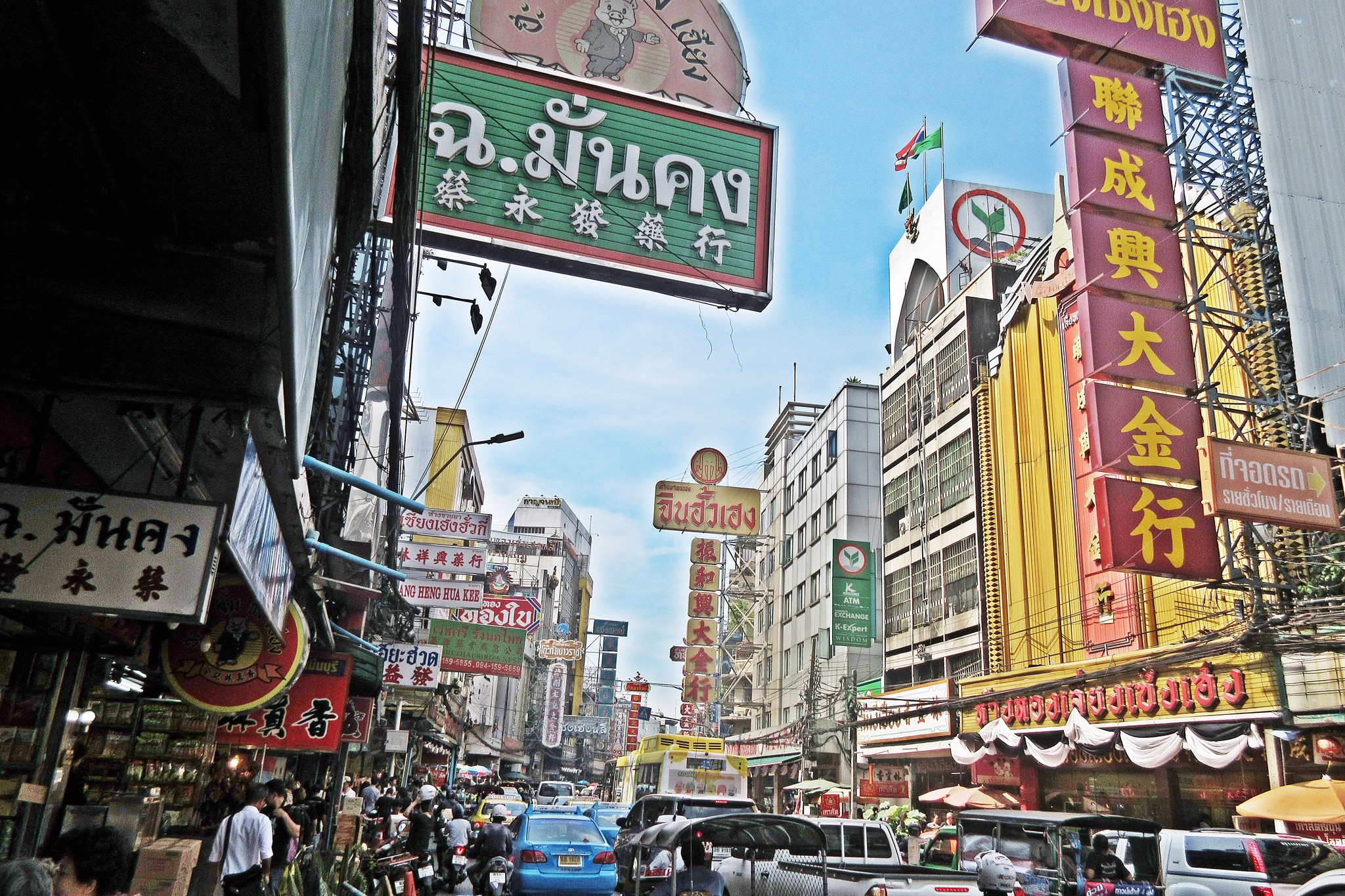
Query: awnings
xmin=747 ymin=754 xmax=801 ymax=768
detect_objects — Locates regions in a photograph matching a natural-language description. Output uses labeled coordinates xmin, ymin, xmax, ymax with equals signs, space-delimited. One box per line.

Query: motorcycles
xmin=620 ymin=815 xmax=830 ymax=896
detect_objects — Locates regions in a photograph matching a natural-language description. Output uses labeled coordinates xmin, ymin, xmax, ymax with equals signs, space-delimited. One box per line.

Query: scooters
xmin=361 ymin=822 xmax=516 ymax=896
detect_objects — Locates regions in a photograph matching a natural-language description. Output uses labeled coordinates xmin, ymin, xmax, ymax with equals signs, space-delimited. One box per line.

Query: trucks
xmin=922 ymin=809 xmax=1164 ymax=895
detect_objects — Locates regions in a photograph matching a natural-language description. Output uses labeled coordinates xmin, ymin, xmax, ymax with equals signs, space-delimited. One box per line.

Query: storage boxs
xmin=332 ymin=796 xmax=363 ymax=850
xmin=129 ymin=838 xmax=202 ymax=896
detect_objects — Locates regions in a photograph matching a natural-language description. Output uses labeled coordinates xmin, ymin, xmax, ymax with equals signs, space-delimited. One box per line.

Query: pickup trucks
xmin=710 ymin=818 xmax=983 ymax=896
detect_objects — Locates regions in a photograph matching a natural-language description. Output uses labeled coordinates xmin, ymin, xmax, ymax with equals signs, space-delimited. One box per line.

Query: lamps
xmin=424 ymin=253 xmax=497 ymax=301
xmin=417 ymin=291 xmax=483 ymax=335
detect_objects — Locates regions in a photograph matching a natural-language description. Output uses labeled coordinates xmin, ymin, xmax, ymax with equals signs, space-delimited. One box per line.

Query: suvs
xmin=615 ymin=792 xmax=763 ymax=848
xmin=1156 ymin=827 xmax=1345 ymax=896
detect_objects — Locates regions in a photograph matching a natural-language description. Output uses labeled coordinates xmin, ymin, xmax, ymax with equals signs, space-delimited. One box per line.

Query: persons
xmin=208 ymin=779 xmax=325 ymax=896
xmin=0 ymin=825 xmax=137 ymax=896
xmin=467 ymin=804 xmax=514 ymax=895
xmin=339 ymin=770 xmax=465 ymax=838
xmin=855 ymin=802 xmax=954 ymax=866
xmin=402 ymin=785 xmax=438 ymax=856
xmin=650 ymin=837 xmax=724 ymax=896
xmin=435 ymin=803 xmax=473 ymax=874
xmin=974 ymin=850 xmax=1016 ymax=896
xmin=1085 ymin=834 xmax=1133 ymax=883
xmin=783 ymin=804 xmax=793 ymax=815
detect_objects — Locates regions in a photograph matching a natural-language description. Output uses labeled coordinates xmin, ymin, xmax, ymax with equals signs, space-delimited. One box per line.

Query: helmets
xmin=974 ymin=849 xmax=1017 ymax=893
xmin=908 ymin=825 xmax=922 ymax=835
xmin=446 ymin=790 xmax=455 ymax=799
xmin=946 ymin=812 xmax=954 ymax=819
xmin=420 ymin=784 xmax=437 ymax=800
xmin=452 ymin=803 xmax=464 ymax=818
xmin=491 ymin=804 xmax=508 ymax=818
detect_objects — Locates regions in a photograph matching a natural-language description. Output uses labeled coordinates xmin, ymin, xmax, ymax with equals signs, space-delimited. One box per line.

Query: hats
xmin=344 ymin=776 xmax=352 ymax=784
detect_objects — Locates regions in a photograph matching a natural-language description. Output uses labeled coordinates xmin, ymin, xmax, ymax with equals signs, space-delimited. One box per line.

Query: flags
xmin=898 ymin=171 xmax=913 ymax=214
xmin=894 ymin=125 xmax=943 ymax=171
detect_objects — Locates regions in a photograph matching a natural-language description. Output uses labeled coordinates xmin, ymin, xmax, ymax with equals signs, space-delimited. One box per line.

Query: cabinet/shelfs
xmin=82 ymin=698 xmax=218 ymax=826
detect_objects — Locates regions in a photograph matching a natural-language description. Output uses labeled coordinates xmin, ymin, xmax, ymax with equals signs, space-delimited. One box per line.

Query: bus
xmin=610 ymin=733 xmax=749 ymax=802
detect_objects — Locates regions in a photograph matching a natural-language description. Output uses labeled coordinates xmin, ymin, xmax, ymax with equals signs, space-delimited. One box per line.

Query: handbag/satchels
xmin=276 ymin=860 xmax=304 ymax=896
xmin=222 ymin=863 xmax=267 ymax=896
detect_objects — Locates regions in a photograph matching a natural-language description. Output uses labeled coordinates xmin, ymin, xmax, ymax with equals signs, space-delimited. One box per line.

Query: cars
xmin=502 ymin=805 xmax=620 ymax=895
xmin=465 ymin=778 xmax=637 ymax=849
xmin=1090 ymin=829 xmax=1157 ymax=878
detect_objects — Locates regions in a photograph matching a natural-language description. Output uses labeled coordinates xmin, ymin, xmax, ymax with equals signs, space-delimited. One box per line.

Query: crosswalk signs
xmin=637 ymin=706 xmax=652 ymax=722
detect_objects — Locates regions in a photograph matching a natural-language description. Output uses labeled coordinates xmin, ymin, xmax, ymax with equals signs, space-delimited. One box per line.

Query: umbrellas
xmin=445 ymin=763 xmax=492 ymax=784
xmin=578 ymin=780 xmax=589 ymax=785
xmin=1236 ymin=774 xmax=1345 ymax=823
xmin=503 ymin=770 xmax=532 ymax=781
xmin=783 ymin=778 xmax=852 ymax=795
xmin=918 ymin=784 xmax=1020 ymax=811
